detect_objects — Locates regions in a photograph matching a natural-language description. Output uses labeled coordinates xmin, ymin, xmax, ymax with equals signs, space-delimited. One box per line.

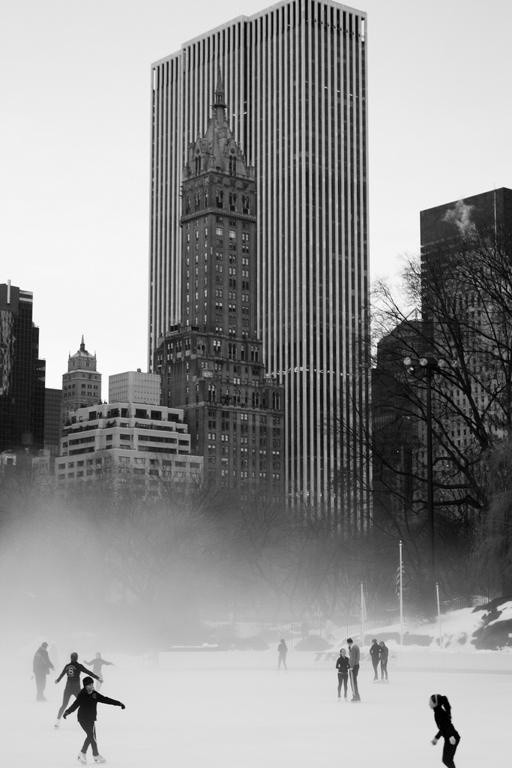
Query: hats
xmin=83 ymin=677 xmax=93 ymax=686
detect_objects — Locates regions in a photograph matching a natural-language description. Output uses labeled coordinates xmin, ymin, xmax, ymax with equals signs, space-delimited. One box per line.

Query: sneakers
xmin=93 ymin=755 xmax=103 ymax=761
xmin=79 ymin=752 xmax=87 ymax=762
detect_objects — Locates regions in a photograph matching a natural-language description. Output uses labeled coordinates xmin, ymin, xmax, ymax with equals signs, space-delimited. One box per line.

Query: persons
xmin=345 ymin=637 xmax=364 ymax=704
xmin=52 ymin=651 xmax=106 ymax=728
xmin=62 ymin=675 xmax=127 ymax=762
xmin=427 ymin=692 xmax=460 ymax=767
xmin=275 ymin=637 xmax=288 ymax=671
xmin=334 ymin=647 xmax=349 ymax=698
xmin=377 ymin=640 xmax=390 ymax=681
xmin=30 ymin=640 xmax=54 ymax=705
xmin=369 ymin=638 xmax=383 ymax=681
xmin=82 ymin=651 xmax=117 ymax=690
